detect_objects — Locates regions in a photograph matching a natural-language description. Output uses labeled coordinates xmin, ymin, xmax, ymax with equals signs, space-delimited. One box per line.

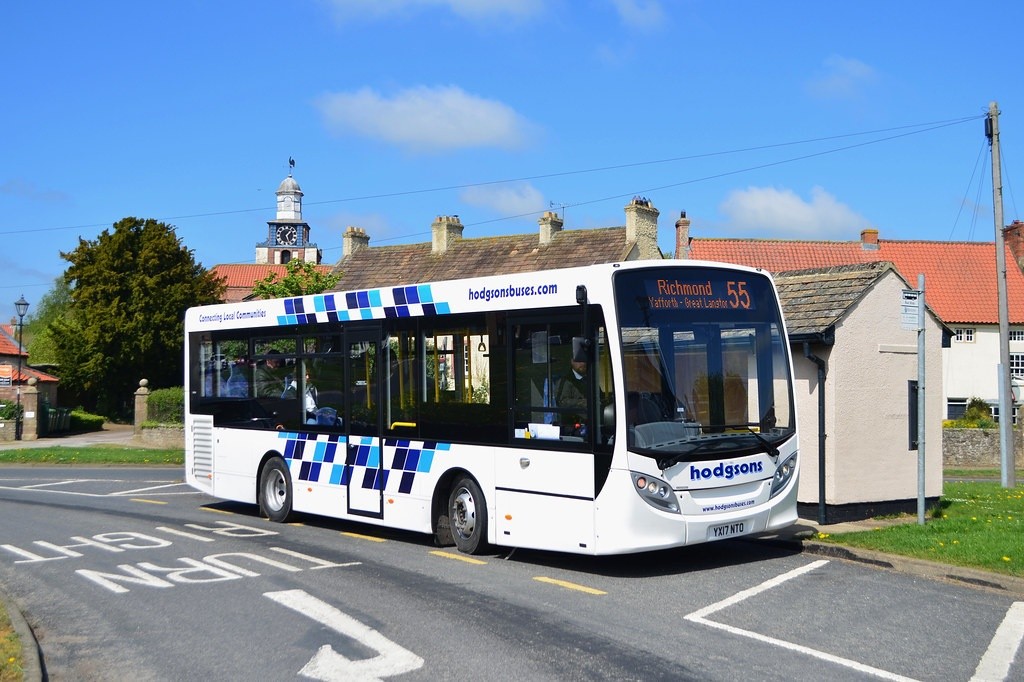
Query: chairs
xmin=205 ymin=353 xmax=561 ymax=439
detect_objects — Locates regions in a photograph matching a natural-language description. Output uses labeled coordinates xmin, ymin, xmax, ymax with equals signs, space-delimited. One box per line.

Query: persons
xmin=235 ymin=354 xmax=250 ymax=365
xmin=282 ymin=368 xmax=321 ymax=421
xmin=256 ymin=350 xmax=286 ymax=398
xmin=554 ymin=356 xmax=604 ymax=424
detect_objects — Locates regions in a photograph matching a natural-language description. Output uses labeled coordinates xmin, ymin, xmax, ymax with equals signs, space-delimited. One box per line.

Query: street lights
xmin=14 ymin=294 xmax=30 ymax=441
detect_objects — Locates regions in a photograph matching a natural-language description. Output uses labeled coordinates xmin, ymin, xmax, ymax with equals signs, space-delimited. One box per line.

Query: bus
xmin=184 ymin=259 xmax=801 ymax=557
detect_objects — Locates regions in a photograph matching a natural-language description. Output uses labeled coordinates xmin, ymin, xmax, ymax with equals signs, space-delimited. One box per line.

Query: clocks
xmin=276 ymin=225 xmax=297 ymax=246
